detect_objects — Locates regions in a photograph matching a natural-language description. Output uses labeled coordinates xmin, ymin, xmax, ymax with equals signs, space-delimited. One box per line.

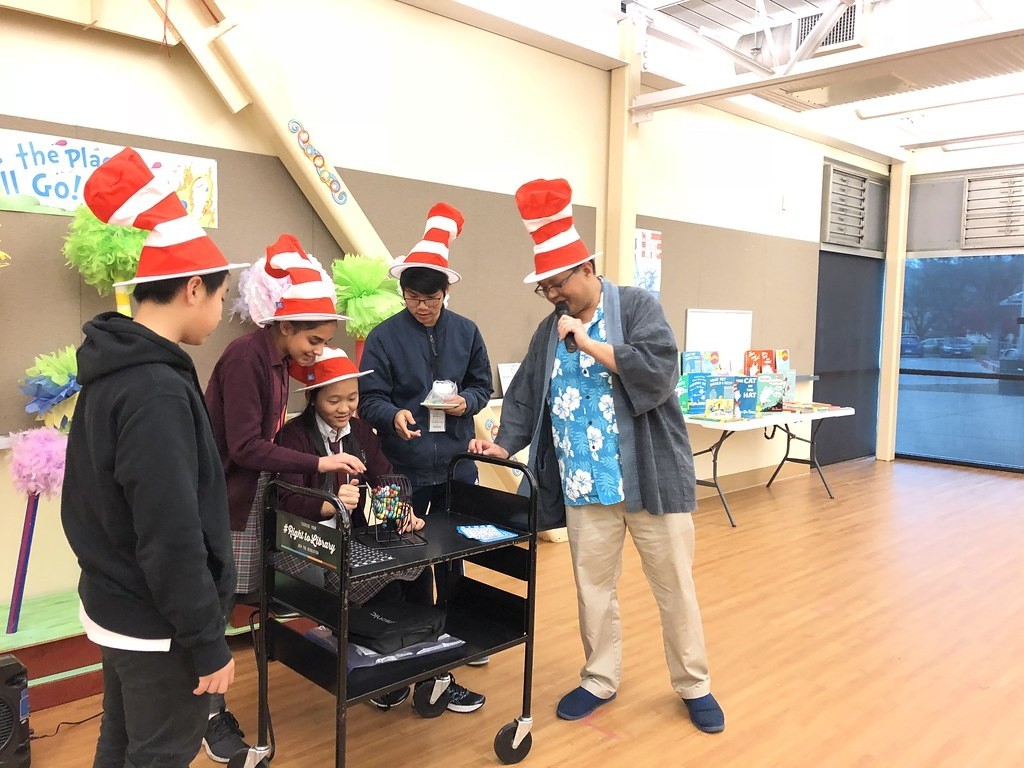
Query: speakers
xmin=0 ymin=653 xmax=31 ymax=768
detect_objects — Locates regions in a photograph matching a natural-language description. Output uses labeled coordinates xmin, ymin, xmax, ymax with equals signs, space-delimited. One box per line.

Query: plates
xmin=419 ymin=403 xmax=461 ymax=409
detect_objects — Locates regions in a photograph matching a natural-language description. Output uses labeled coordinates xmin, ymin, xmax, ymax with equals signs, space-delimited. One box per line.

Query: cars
xmin=922 ymin=339 xmax=945 ymax=354
xmin=901 ymin=333 xmax=924 ymax=357
xmin=939 ymin=337 xmax=975 ymax=358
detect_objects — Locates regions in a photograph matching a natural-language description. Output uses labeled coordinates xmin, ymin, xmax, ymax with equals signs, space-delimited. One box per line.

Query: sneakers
xmin=203 ymin=711 xmax=245 ymax=762
xmin=683 ymin=691 xmax=724 ymax=733
xmin=413 ymin=678 xmax=484 ymax=714
xmin=371 ymin=685 xmax=410 ymax=710
xmin=557 ymin=686 xmax=617 ymax=720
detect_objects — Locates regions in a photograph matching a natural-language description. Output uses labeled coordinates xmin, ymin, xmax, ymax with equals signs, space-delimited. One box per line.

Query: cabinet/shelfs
xmin=226 ymin=450 xmax=542 ymax=768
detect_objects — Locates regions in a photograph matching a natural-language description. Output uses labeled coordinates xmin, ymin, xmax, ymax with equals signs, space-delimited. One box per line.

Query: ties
xmin=328 ymin=436 xmax=347 ymax=494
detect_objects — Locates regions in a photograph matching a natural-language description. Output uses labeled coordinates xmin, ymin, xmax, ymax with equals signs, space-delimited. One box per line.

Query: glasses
xmin=534 ymin=267 xmax=577 ymax=298
xmin=403 ymin=290 xmax=444 ymax=307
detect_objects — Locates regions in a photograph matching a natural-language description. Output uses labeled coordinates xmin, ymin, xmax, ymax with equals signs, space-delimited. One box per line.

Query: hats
xmin=289 ymin=344 xmax=375 ymax=393
xmin=389 ymin=203 xmax=464 ymax=285
xmin=516 ymin=179 xmax=604 ymax=284
xmin=83 ymin=147 xmax=250 ymax=286
xmin=258 ymin=235 xmax=355 ymax=326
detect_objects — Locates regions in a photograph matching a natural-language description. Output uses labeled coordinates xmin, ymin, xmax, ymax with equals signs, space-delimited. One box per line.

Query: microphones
xmin=555 ymin=301 xmax=577 ymax=354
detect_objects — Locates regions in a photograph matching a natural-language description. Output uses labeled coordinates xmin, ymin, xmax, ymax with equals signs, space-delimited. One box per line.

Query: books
xmin=675 ymin=348 xmax=840 ymax=423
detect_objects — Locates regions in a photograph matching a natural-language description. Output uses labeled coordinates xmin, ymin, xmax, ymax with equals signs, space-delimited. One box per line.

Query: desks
xmin=676 ymin=401 xmax=854 ymax=527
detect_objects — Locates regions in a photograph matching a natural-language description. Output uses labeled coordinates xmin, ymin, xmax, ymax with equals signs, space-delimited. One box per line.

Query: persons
xmin=60 ymin=271 xmax=236 ymax=768
xmin=274 ymin=376 xmax=486 ymax=713
xmin=202 ymin=319 xmax=367 ymax=763
xmin=358 ymin=267 xmax=493 ymax=665
xmin=467 ymin=260 xmax=725 ymax=733
